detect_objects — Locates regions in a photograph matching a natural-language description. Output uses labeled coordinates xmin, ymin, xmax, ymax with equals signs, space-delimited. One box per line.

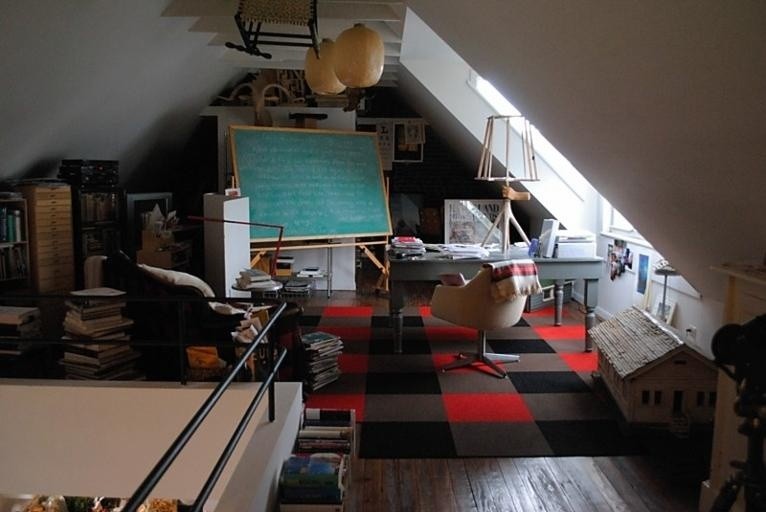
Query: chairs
xmin=431 ymin=259 xmax=543 ymax=377
xmin=101 ymin=251 xmax=304 ymax=343
xmin=138 ymin=263 xmax=215 ymax=298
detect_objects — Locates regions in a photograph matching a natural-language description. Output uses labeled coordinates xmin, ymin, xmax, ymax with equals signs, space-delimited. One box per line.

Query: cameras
xmin=711 ymin=313 xmax=766 ymax=407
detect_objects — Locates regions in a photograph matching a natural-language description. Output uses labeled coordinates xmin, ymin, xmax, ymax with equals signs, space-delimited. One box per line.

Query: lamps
xmin=335 ymin=24 xmax=384 ymax=87
xmin=306 ymin=38 xmax=347 ymax=95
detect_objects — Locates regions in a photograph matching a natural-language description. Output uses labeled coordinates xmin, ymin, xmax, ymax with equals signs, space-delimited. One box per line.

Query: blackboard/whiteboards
xmin=228 ymin=123 xmax=393 ymax=242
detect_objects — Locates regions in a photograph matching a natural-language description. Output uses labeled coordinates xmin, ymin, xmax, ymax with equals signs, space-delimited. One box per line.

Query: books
xmin=235 ymin=269 xmax=273 ymax=289
xmin=386 ymin=235 xmax=489 ymax=260
xmin=0 ymin=189 xmax=142 ymax=381
xmin=296 ymin=267 xmax=325 ymax=277
xmin=301 ymin=330 xmax=345 ymax=392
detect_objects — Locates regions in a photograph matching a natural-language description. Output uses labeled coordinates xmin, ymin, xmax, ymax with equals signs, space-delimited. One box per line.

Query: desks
xmin=388 ymin=244 xmax=604 ymax=353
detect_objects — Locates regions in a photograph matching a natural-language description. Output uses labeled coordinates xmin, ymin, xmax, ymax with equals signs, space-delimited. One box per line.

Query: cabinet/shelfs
xmin=0 ymin=199 xmax=31 ymax=288
xmin=70 ymin=188 xmax=125 ymax=276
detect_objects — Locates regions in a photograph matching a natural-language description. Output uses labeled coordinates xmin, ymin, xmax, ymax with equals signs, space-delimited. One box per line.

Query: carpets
xmin=275 ymin=304 xmax=653 ymax=460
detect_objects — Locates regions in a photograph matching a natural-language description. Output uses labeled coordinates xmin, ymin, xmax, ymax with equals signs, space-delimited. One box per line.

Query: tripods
xmin=709 ymin=461 xmax=766 ymax=512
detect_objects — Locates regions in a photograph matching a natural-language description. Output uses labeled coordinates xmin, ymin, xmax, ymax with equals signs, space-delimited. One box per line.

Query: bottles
xmin=528 ymin=238 xmax=538 ymax=257
